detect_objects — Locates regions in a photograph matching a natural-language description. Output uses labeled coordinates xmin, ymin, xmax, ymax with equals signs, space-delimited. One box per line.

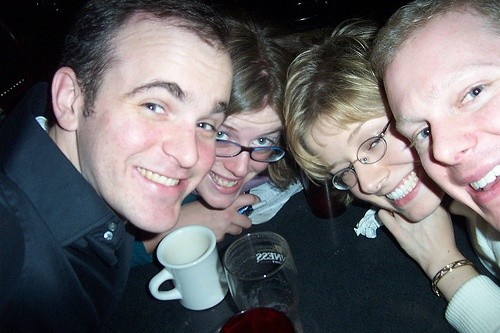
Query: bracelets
xmin=430 ymin=258 xmax=474 ymax=300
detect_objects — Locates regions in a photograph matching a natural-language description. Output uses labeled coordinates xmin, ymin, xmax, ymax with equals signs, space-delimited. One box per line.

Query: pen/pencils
xmin=237 ymin=200 xmax=267 ymax=214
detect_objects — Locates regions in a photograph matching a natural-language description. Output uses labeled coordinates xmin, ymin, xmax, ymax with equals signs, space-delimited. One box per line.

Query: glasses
xmin=332 ymin=118 xmax=394 ymax=190
xmin=215 ymin=138 xmax=285 ymax=163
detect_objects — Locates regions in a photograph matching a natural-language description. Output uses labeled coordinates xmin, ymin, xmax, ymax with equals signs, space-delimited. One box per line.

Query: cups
xmin=223 ymin=231 xmax=301 ymax=316
xmin=148 ymin=224 xmax=229 ymax=310
xmin=299 ymin=169 xmax=347 ymax=220
xmin=212 ymin=306 xmax=299 ymax=333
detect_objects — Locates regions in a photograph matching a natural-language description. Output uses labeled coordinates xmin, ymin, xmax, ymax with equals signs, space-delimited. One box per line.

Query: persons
xmin=123 ymin=19 xmax=286 ymax=255
xmin=0 ymin=0 xmax=235 ymax=333
xmin=375 ymin=0 xmax=500 ymax=332
xmin=283 ymin=20 xmax=500 ymax=276
